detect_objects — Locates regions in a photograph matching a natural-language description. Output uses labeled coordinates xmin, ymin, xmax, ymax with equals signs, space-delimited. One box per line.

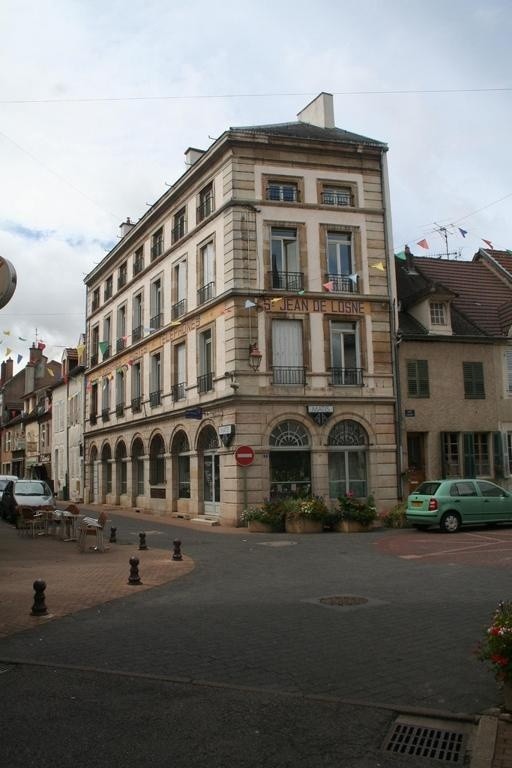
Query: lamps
xmin=249 ymin=347 xmax=262 ymax=371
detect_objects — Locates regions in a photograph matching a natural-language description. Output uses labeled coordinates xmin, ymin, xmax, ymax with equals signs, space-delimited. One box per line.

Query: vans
xmin=0 ymin=474 xmax=18 ymax=502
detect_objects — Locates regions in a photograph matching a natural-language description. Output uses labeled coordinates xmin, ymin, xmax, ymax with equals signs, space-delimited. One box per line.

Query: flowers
xmin=333 ymin=490 xmax=388 ymax=522
xmin=240 ymin=493 xmax=331 ymax=533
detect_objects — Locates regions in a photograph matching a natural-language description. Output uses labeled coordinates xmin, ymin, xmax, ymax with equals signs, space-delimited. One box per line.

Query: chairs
xmin=17 ymin=504 xmax=112 ymax=552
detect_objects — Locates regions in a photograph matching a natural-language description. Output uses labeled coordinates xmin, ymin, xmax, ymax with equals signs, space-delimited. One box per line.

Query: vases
xmin=336 ymin=518 xmax=383 ymax=532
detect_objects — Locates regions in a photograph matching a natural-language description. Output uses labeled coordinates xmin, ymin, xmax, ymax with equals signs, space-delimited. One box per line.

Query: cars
xmin=405 ymin=477 xmax=512 ymax=532
xmin=0 ymin=478 xmax=57 ymax=524
